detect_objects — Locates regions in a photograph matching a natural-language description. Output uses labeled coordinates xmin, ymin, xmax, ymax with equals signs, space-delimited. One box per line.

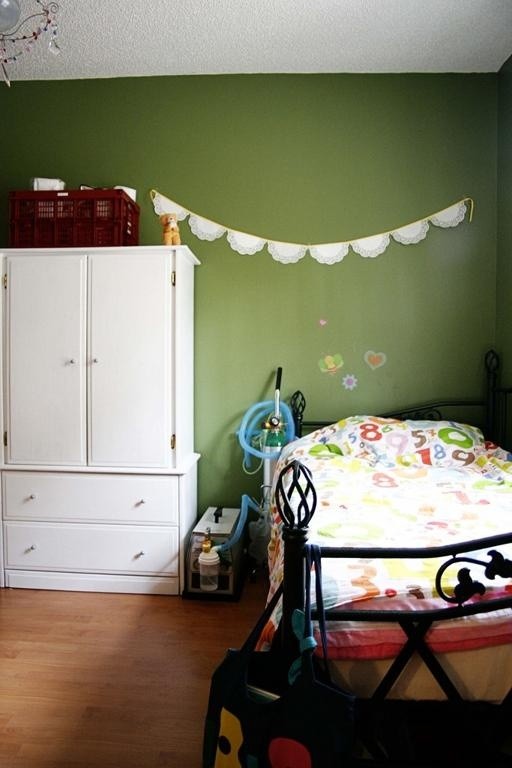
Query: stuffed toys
xmin=161 ymin=212 xmax=182 ymax=246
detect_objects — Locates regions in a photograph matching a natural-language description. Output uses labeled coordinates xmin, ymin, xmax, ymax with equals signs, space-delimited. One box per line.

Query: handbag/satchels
xmin=203 ymin=648 xmax=363 ymax=767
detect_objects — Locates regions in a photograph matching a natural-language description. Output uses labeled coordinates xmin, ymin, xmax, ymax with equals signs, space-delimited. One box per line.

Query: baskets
xmin=6 ymin=190 xmax=140 ymax=246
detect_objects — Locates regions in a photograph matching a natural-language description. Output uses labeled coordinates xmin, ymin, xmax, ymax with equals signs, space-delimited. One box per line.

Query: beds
xmin=274 ymin=348 xmax=510 ymax=766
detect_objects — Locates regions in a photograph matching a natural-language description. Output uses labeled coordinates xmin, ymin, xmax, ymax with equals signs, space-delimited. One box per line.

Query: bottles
xmin=263 ymin=428 xmax=285 ymax=457
xmin=198 ymin=550 xmax=221 ymax=592
